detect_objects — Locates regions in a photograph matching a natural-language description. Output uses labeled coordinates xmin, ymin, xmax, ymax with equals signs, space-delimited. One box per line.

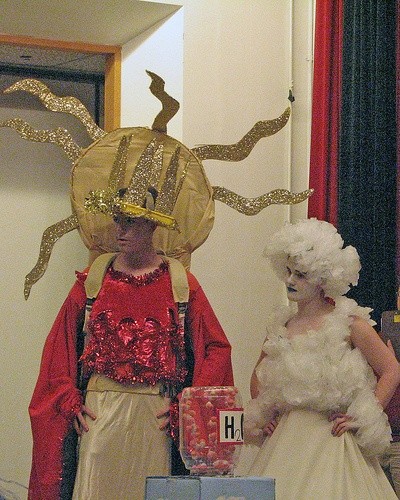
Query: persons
xmin=241 ymin=217 xmax=399 ymax=500
xmin=26 ymin=189 xmax=236 ymax=500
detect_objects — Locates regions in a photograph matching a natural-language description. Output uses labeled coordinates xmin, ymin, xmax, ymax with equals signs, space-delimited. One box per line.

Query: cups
xmin=179 ymin=386 xmax=244 ymax=476
